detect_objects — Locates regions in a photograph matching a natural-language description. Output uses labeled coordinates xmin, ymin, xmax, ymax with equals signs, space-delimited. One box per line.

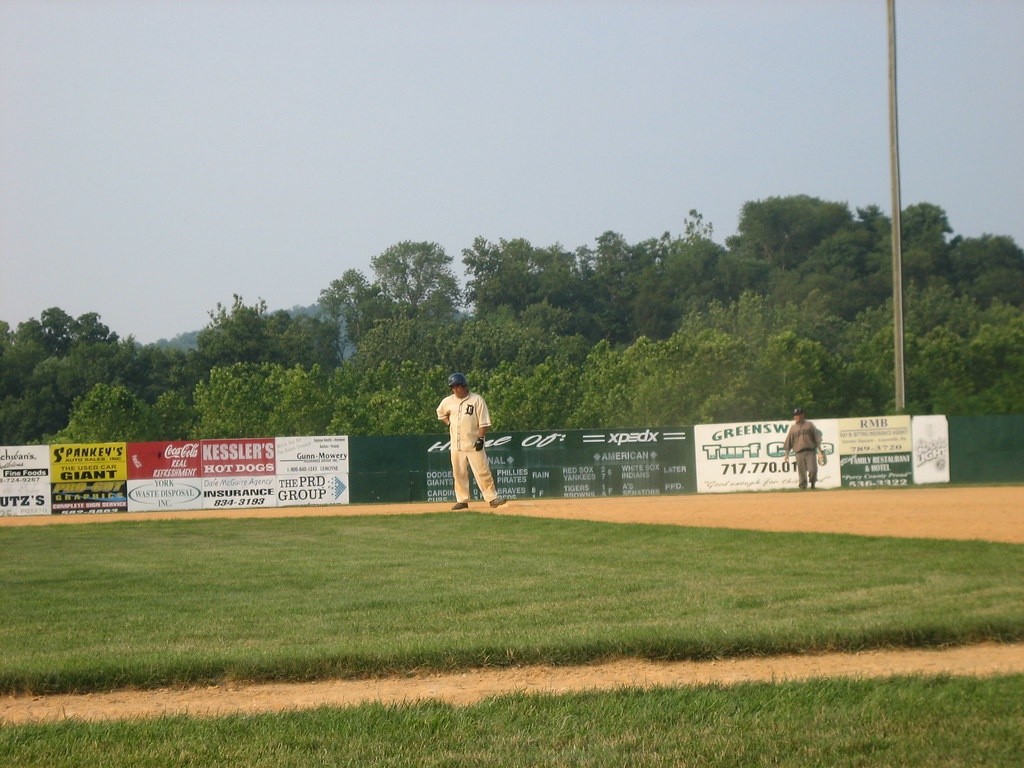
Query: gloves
xmin=473 ymin=437 xmax=485 ymax=451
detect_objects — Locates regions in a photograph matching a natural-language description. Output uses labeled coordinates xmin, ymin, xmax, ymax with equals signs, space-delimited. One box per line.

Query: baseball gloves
xmin=817 ymin=451 xmax=827 ymax=466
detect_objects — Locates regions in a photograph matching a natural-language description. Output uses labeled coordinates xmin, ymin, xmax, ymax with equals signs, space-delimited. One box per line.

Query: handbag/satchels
xmin=818 ymin=449 xmax=827 ymax=466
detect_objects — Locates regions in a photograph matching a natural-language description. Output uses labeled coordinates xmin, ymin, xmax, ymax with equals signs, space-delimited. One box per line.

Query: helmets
xmin=448 ymin=372 xmax=467 ymax=387
xmin=793 ymin=407 xmax=803 ymax=416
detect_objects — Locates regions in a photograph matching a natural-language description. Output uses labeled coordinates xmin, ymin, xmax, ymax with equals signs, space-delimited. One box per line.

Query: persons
xmin=784 ymin=408 xmax=827 ymax=489
xmin=435 ymin=373 xmax=507 ymax=510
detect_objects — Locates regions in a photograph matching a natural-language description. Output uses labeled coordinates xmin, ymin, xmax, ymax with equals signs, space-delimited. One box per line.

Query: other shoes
xmin=489 ymin=497 xmax=507 ymax=508
xmin=452 ymin=502 xmax=469 ymax=510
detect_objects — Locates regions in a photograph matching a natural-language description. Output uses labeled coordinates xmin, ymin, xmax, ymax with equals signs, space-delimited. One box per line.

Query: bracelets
xmin=479 ymin=437 xmax=484 ymax=438
